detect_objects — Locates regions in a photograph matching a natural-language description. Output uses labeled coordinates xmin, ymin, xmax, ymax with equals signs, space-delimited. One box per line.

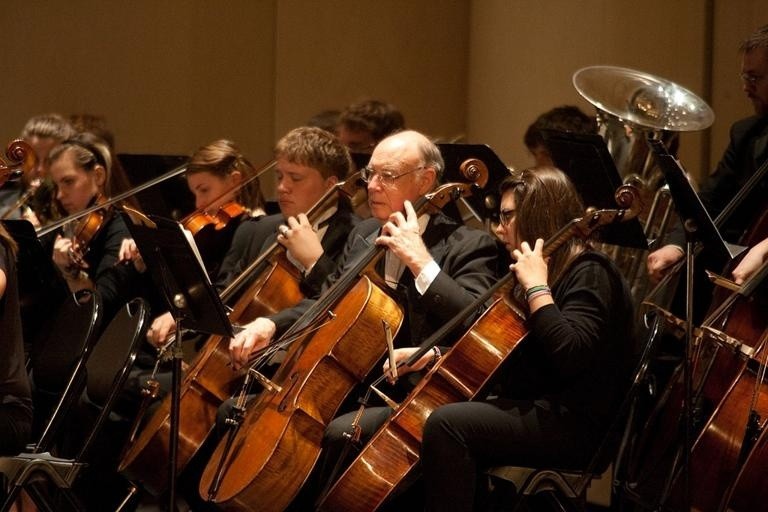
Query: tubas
xmin=573 ymin=65 xmax=715 ymax=340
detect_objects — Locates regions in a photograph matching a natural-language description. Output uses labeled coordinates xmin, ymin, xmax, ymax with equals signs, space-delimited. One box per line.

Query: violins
xmin=63 ymin=193 xmax=113 ymax=280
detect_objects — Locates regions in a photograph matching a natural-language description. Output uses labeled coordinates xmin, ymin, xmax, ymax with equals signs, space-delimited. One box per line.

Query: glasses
xmin=736 ymin=70 xmax=766 ymax=85
xmin=498 ymin=208 xmax=519 ymax=225
xmin=360 ymin=164 xmax=425 ymax=184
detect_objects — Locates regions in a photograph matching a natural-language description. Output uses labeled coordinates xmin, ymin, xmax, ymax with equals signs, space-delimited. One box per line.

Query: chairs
xmin=0 ymin=287 xmax=108 ymax=511
xmin=2 ymin=288 xmax=156 ymax=510
xmin=486 ymin=305 xmax=664 ymax=510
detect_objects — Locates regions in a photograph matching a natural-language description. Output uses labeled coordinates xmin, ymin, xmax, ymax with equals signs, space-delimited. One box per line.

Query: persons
xmin=68 ymin=113 xmax=142 ymax=214
xmin=339 ymin=100 xmax=408 ymax=166
xmin=519 ymin=104 xmax=595 ymax=166
xmin=12 ymin=114 xmax=76 ymax=192
xmin=28 ymin=126 xmax=162 ymax=345
xmin=223 ymin=126 xmax=511 ymax=512
xmin=644 ymin=22 xmax=767 ymax=326
xmin=0 ymin=221 xmax=34 ymax=461
xmin=307 ymin=110 xmax=342 ymax=137
xmin=722 ymin=235 xmax=768 ymax=290
xmin=142 ymin=125 xmax=359 ymax=506
xmin=379 ymin=166 xmax=638 ymax=512
xmin=112 ymin=139 xmax=270 ymax=293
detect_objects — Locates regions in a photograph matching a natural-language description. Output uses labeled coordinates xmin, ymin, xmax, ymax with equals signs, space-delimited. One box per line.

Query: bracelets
xmin=524 ymin=285 xmax=550 ymax=300
xmin=526 ymin=288 xmax=552 ymax=304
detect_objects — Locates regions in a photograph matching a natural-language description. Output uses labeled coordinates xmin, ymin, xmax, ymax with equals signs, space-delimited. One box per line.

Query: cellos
xmin=625 ymin=210 xmax=766 ymax=502
xmin=309 ymin=172 xmax=643 ymax=511
xmin=656 ymin=328 xmax=768 ymax=510
xmin=118 ymin=170 xmax=368 ymax=502
xmin=198 ymin=157 xmax=489 ymax=511
